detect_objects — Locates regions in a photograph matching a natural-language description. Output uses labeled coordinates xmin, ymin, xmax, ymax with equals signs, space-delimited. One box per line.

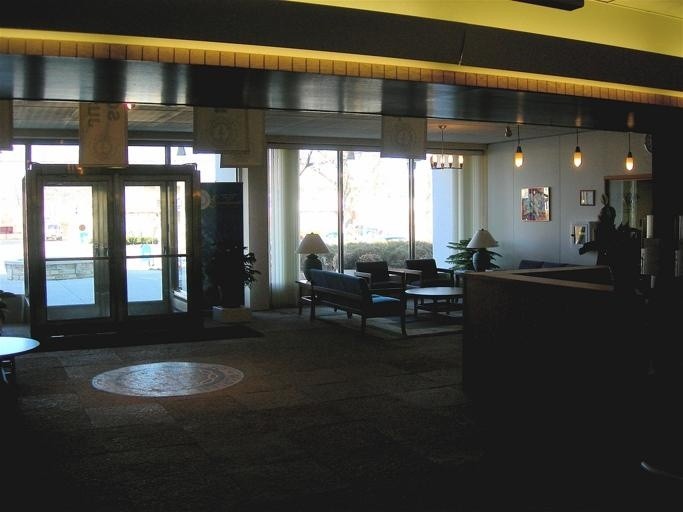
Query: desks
xmin=295 ymin=280 xmax=338 ymax=320
xmin=455 ymin=269 xmax=495 ymax=303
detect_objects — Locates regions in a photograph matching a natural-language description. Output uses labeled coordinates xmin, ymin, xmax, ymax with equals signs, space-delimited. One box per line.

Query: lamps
xmin=625 ymin=130 xmax=634 ymax=171
xmin=514 ymin=122 xmax=523 ymax=168
xmin=294 ymin=232 xmax=329 ymax=281
xmin=466 ymin=228 xmax=498 ymax=273
xmin=430 ymin=124 xmax=464 ymax=170
xmin=573 ymin=126 xmax=582 ymax=168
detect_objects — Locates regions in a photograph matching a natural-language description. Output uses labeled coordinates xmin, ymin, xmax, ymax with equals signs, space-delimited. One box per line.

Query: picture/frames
xmin=579 ymin=190 xmax=595 ymax=207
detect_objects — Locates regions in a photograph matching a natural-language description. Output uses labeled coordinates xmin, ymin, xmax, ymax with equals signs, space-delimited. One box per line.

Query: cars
xmin=46 ymin=223 xmax=64 ymax=241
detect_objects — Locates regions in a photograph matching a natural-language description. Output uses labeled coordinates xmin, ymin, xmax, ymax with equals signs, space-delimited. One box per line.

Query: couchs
xmin=309 ymin=268 xmax=406 ymax=336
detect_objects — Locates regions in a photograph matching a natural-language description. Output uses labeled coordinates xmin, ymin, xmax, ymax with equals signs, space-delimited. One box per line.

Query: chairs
xmin=353 ymin=258 xmax=454 ymax=311
xmin=515 ymin=259 xmax=584 ymax=269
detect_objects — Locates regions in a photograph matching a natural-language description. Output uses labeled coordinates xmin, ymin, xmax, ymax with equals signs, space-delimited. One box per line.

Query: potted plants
xmin=200 ymin=237 xmax=259 ymax=309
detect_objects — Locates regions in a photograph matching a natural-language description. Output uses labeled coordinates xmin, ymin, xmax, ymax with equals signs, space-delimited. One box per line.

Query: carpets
xmin=29 ymin=312 xmax=265 ymax=353
xmin=271 ymin=292 xmax=463 ymax=342
